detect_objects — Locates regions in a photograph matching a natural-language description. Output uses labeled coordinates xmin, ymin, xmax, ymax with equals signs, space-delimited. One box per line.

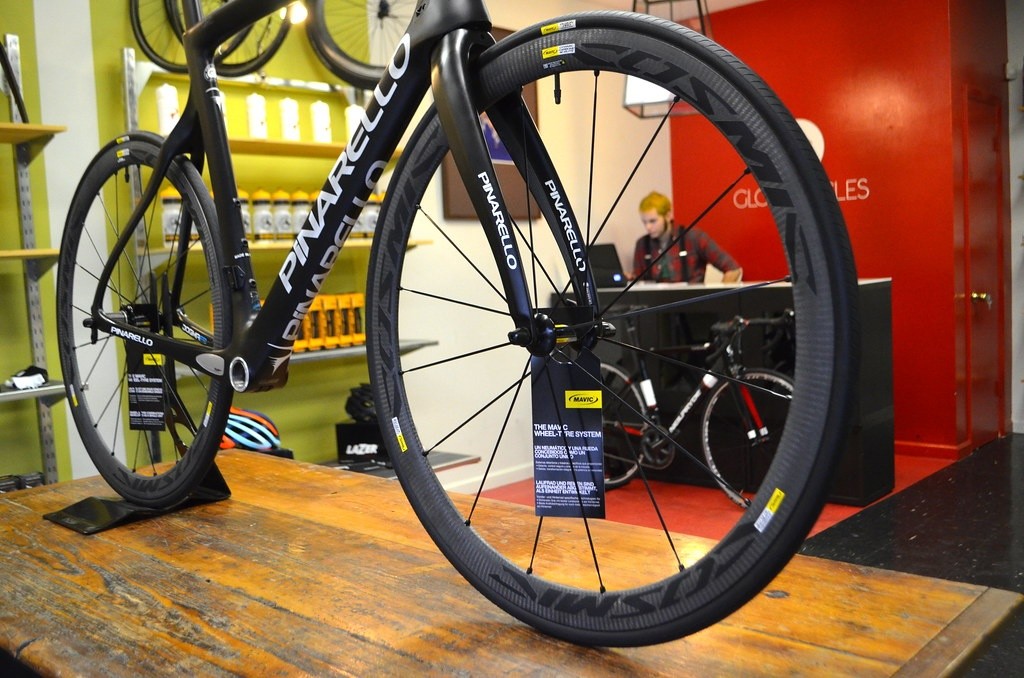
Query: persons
xmin=624 ymin=191 xmax=744 ymax=283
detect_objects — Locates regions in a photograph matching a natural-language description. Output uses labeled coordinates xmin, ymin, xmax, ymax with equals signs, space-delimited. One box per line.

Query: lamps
xmin=623 ymin=0 xmax=715 ymax=117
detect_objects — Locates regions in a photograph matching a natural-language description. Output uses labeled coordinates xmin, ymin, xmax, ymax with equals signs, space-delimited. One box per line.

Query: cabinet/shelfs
xmin=123 ymin=48 xmax=482 ymax=481
xmin=0 ymin=33 xmax=88 ymax=489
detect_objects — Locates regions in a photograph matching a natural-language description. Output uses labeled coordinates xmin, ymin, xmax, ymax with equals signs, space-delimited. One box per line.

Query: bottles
xmin=310 ymin=100 xmax=331 ymax=145
xmin=160 ymin=186 xmax=385 ymax=241
xmin=345 ymin=104 xmax=364 ymax=140
xmin=247 ymin=93 xmax=267 ymax=139
xmin=157 ymin=84 xmax=180 ymax=138
xmin=210 ymin=292 xmax=365 ymax=353
xmin=279 ymin=97 xmax=299 ymax=141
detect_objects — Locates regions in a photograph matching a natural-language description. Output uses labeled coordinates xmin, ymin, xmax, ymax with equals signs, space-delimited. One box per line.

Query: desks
xmin=795 ymin=430 xmax=1024 ymax=678
xmin=0 ymin=446 xmax=1018 ymax=678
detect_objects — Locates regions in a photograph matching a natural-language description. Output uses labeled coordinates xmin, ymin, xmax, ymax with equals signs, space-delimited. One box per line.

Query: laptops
xmin=584 ymin=243 xmax=654 ymax=288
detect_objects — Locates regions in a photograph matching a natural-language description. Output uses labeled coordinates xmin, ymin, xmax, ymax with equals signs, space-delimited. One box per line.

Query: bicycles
xmin=55 ymin=0 xmax=857 ymax=645
xmin=597 ymin=308 xmax=797 ymax=511
